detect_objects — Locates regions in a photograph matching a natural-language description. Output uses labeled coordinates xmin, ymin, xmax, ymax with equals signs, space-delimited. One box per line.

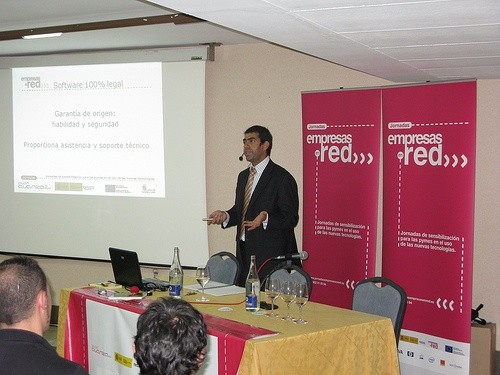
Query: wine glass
xmin=195 ymin=265 xmax=210 ymax=301
xmin=279 ymin=281 xmax=298 ymax=321
xmin=265 ymin=278 xmax=282 ymax=318
xmin=292 ymin=284 xmax=309 ymax=325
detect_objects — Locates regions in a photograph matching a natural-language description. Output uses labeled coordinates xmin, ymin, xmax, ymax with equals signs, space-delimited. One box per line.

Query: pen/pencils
xmin=128 ymin=292 xmax=142 ymax=296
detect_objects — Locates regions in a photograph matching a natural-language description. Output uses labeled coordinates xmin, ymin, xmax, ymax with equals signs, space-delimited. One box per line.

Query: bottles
xmin=168 ymin=247 xmax=183 ymax=300
xmin=245 ymin=255 xmax=261 ymax=313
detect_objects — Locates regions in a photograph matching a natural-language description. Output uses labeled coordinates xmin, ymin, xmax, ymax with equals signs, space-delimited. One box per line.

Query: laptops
xmin=109 ymin=247 xmax=169 ymax=291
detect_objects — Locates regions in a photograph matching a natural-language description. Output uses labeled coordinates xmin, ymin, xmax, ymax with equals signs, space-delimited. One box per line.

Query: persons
xmin=0 ymin=254 xmax=85 ymax=375
xmin=132 ymin=297 xmax=207 ymax=375
xmin=208 ymin=125 xmax=303 ymax=293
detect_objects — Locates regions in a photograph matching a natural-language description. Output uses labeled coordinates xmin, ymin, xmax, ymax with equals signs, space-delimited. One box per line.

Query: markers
xmin=202 ymin=218 xmax=213 ymax=221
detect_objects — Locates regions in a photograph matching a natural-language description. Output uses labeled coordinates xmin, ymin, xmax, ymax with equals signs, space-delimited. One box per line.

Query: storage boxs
xmin=470 ymin=320 xmax=496 ymax=375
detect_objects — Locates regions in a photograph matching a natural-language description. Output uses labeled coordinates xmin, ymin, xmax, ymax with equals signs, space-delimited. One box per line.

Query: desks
xmin=56 ymin=273 xmax=402 ymax=375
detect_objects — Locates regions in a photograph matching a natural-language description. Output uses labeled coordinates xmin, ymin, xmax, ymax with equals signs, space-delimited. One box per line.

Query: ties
xmin=239 ymin=167 xmax=258 ymax=235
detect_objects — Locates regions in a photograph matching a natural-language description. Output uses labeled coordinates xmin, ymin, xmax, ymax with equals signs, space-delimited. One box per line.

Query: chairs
xmin=205 ymin=251 xmax=241 ymax=285
xmin=261 ymin=261 xmax=314 ymax=300
xmin=351 ymin=275 xmax=407 ymax=349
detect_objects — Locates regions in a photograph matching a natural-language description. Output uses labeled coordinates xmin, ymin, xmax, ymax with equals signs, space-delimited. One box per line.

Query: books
xmin=109 ymin=293 xmax=146 ymax=300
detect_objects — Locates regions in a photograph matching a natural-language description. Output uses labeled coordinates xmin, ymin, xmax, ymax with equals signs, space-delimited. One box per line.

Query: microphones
xmin=239 ymin=152 xmax=245 ymax=161
xmin=271 ymin=251 xmax=308 ymax=260
